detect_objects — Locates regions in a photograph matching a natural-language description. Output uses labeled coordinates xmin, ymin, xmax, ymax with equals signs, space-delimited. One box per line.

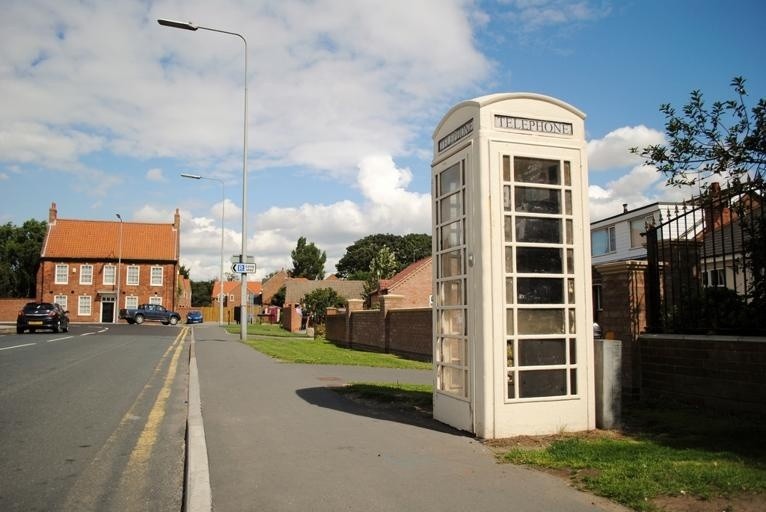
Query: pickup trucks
xmin=119 ymin=304 xmax=181 ymax=325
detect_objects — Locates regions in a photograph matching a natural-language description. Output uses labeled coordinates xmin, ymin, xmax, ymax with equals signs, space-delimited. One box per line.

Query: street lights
xmin=116 ymin=213 xmax=124 ymax=323
xmin=158 ymin=16 xmax=248 ymax=341
xmin=181 ymin=174 xmax=225 ymax=327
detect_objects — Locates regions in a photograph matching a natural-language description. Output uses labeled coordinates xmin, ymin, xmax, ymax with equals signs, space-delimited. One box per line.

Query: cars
xmin=17 ymin=302 xmax=69 ymax=334
xmin=186 ymin=310 xmax=203 ymax=324
